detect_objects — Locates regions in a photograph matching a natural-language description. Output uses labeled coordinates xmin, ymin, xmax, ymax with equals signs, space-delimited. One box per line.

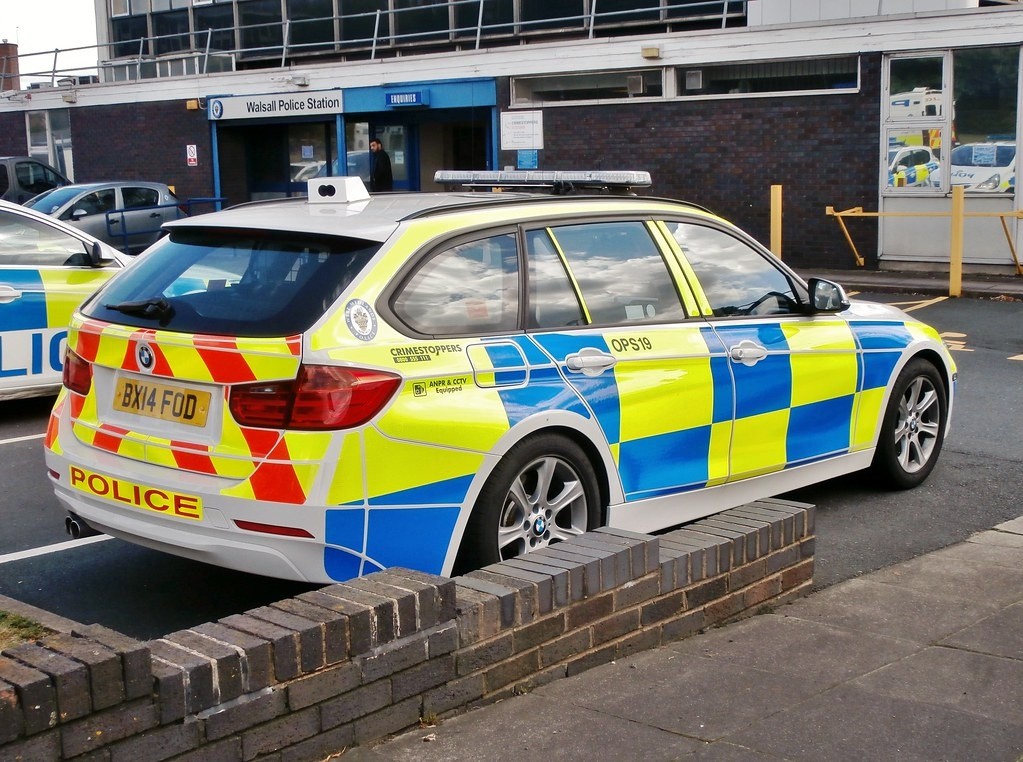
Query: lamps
xmin=62 ymin=90 xmax=78 ymax=104
xmin=292 ymin=76 xmax=310 ymax=87
xmin=640 ymin=41 xmax=664 ymax=60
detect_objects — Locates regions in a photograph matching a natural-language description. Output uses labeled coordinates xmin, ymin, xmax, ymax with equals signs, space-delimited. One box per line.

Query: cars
xmin=291 ymin=150 xmax=371 ymax=191
xmin=0 ymin=200 xmax=244 ymax=403
xmin=0 ymin=181 xmax=190 ymax=261
xmin=887 ymin=88 xmax=1016 ymax=195
xmin=46 ymin=168 xmax=959 ymax=586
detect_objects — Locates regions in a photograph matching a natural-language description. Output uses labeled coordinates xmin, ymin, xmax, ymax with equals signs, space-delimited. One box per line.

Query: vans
xmin=0 ymin=156 xmax=74 ymax=206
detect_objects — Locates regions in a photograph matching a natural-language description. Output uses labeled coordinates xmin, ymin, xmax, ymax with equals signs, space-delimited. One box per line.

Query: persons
xmin=370 ymin=138 xmax=393 ymax=192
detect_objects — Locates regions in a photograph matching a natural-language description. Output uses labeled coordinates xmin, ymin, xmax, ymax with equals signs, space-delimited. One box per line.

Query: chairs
xmin=101 ymin=194 xmax=114 ymax=204
xmin=288 ymin=262 xmax=323 ymax=301
xmin=87 ymin=195 xmax=99 ymax=206
